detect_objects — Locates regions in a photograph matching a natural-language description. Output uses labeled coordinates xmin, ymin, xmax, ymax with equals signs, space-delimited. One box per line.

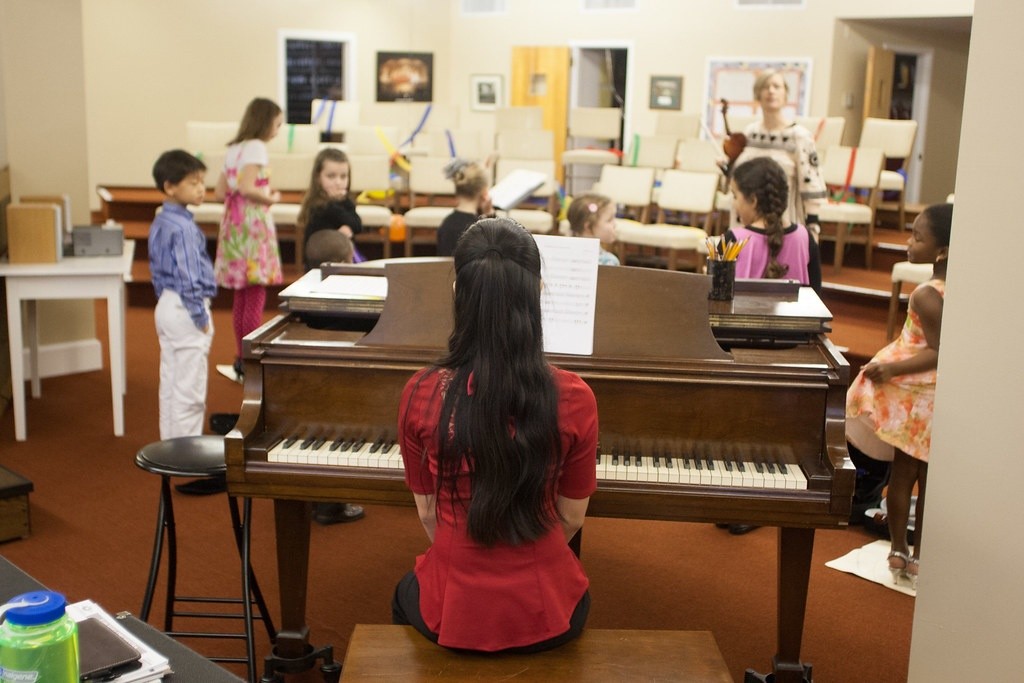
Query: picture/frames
xmin=650 ymin=77 xmax=681 ymax=108
xmin=701 ymin=59 xmax=812 ymax=145
xmin=469 ymin=75 xmax=502 ymax=110
xmin=376 ymin=52 xmax=434 ymax=103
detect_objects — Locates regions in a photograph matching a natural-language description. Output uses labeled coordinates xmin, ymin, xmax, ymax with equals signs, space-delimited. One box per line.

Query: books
xmin=75 ymin=618 xmax=142 ymax=683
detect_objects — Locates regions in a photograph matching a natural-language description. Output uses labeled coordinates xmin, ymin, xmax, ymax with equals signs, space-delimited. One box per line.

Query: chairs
xmin=185 ymin=105 xmax=955 ymax=343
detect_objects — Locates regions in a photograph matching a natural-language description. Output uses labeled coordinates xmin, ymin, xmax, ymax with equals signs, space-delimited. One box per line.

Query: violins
xmin=720 ymin=99 xmax=748 ymax=158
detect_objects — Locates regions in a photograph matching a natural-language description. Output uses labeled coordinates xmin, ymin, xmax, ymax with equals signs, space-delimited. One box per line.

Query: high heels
xmin=905 ymin=558 xmax=919 ymax=591
xmin=887 ymin=550 xmax=911 ymax=584
xmin=234 ymin=358 xmax=246 ymax=381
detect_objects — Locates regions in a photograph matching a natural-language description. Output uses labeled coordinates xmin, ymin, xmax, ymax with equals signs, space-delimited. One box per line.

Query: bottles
xmin=0 ymin=590 xmax=80 ymax=683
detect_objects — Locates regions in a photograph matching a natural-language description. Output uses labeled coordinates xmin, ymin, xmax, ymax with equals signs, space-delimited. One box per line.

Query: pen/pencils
xmin=704 ymin=233 xmax=753 ymax=260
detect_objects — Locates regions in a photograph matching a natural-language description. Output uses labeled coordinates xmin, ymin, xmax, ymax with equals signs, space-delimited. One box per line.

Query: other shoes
xmin=316 ymin=505 xmax=363 ymax=523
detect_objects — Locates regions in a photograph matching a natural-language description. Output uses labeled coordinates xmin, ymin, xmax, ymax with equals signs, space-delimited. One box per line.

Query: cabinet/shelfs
xmin=97 ymin=188 xmax=490 ymax=286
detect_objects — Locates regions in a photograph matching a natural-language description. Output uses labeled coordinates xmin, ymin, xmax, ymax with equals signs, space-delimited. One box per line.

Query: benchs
xmin=338 ymin=624 xmax=734 ymax=683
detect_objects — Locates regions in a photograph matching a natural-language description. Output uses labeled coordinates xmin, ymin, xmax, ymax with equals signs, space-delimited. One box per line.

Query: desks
xmin=0 ymin=238 xmax=137 ymax=444
xmin=0 ymin=554 xmax=250 ymax=683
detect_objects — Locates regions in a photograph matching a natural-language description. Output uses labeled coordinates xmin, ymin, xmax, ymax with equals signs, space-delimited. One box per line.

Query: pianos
xmin=219 ymin=241 xmax=857 ymax=683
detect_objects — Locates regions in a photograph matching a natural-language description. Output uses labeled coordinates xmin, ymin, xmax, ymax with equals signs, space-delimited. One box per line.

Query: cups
xmin=706 ymin=256 xmax=736 ymax=301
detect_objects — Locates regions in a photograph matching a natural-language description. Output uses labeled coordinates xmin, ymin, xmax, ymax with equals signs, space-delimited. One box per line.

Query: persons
xmin=567 ymin=194 xmax=621 ymax=267
xmin=436 ymin=159 xmax=499 ymax=256
xmin=148 ymin=149 xmax=218 ymax=440
xmin=288 ymin=230 xmax=366 ymax=524
xmin=295 ymin=150 xmax=363 ymax=272
xmin=392 ymin=219 xmax=599 ymax=658
xmin=846 ymin=204 xmax=955 ymax=591
xmin=214 ymin=98 xmax=283 ymax=384
xmin=723 ymin=68 xmax=828 ymax=255
xmin=707 ymin=156 xmax=823 ymax=297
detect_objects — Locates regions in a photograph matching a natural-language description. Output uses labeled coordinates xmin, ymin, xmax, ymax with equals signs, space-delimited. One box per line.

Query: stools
xmin=135 ymin=437 xmax=275 ymax=683
